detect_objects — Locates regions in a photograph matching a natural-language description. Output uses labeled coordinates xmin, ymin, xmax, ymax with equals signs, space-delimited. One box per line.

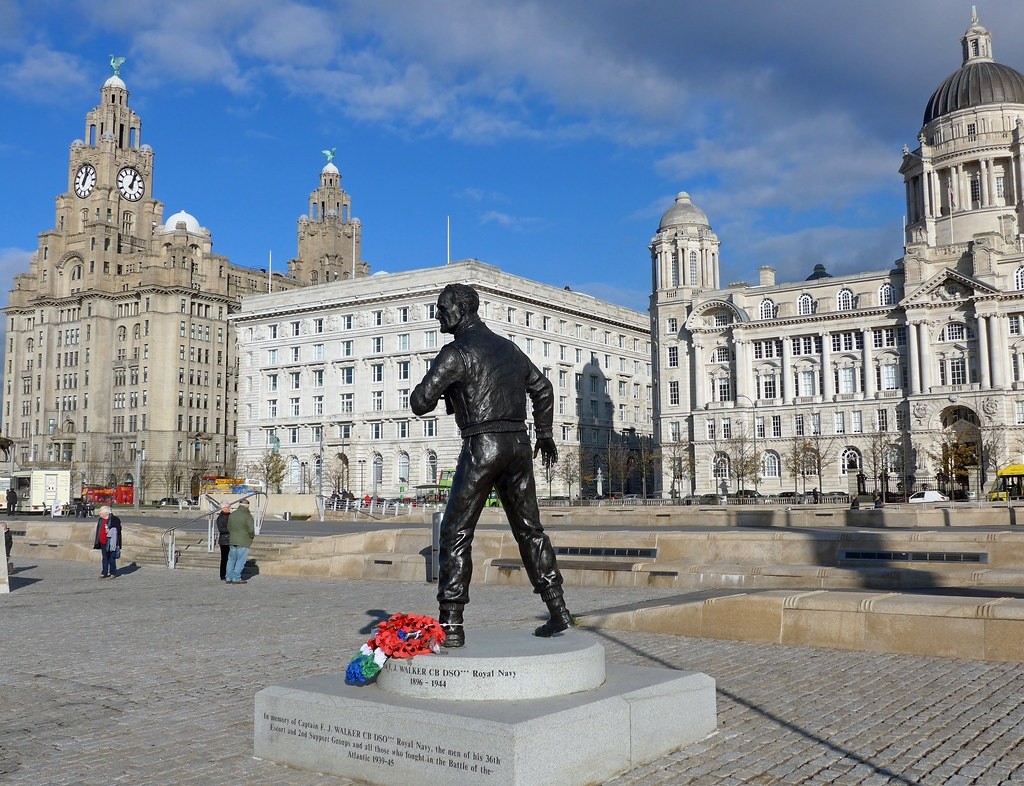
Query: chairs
xmin=42 ymin=500 xmax=95 ymax=519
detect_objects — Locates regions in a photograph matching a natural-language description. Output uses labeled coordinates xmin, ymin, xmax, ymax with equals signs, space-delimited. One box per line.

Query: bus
xmin=439 ymin=468 xmax=504 ymax=507
xmin=988 ymin=465 xmax=1024 ymax=502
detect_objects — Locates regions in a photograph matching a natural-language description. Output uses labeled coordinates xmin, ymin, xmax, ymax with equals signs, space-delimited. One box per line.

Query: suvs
xmin=736 ymin=490 xmax=768 ymax=504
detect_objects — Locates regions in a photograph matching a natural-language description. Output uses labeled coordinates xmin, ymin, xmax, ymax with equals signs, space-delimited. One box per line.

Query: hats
xmin=239 ymin=499 xmax=250 ymax=504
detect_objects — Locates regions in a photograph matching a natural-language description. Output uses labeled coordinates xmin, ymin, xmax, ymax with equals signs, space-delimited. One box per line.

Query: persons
xmin=6 ymin=488 xmax=17 ymax=516
xmin=76 ymin=498 xmax=85 ymax=518
xmin=217 ymin=499 xmax=255 ymax=584
xmin=851 ymin=495 xmax=859 ymax=510
xmin=1007 ymin=481 xmax=1015 ymax=490
xmin=0 ymin=522 xmax=12 ymax=562
xmin=874 ymin=496 xmax=883 ymax=509
xmin=879 ymin=468 xmax=891 ymax=493
xmin=422 ymin=493 xmax=442 ymax=507
xmin=856 ymin=470 xmax=869 ymax=495
xmin=330 ymin=489 xmax=380 ymax=510
xmin=93 ymin=506 xmax=122 ymax=578
xmin=410 ymin=283 xmax=575 ymax=648
xmin=935 ymin=469 xmax=946 ymax=490
xmin=813 ymin=487 xmax=818 ymax=504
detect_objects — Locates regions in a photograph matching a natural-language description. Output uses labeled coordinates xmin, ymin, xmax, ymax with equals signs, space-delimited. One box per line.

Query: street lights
xmin=737 ymin=394 xmax=758 ymax=504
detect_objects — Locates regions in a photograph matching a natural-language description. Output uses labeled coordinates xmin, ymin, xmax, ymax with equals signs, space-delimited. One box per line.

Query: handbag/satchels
xmin=112 ymin=549 xmax=120 ymax=559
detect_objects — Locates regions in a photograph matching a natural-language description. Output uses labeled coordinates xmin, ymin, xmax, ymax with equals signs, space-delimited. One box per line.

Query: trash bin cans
xmin=719 ymin=495 xmax=728 ymax=505
xmin=432 ymin=511 xmax=445 ymax=586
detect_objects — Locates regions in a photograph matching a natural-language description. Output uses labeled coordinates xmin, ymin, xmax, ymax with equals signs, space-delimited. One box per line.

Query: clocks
xmin=116 ymin=167 xmax=145 ymax=201
xmin=74 ymin=164 xmax=97 ymax=200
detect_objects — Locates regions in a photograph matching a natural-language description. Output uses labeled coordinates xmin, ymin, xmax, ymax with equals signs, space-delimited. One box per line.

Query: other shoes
xmin=100 ymin=574 xmax=107 ymax=579
xmin=110 ymin=574 xmax=116 ymax=578
xmin=226 ymin=580 xmax=248 ymax=584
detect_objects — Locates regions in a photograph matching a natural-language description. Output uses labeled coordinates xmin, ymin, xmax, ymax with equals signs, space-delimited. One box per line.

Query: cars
xmin=160 ymin=498 xmax=197 ymax=505
xmin=769 ymin=491 xmax=849 ymax=504
xmin=683 ymin=493 xmax=720 ymax=505
xmin=536 ymin=492 xmax=656 ymax=506
xmin=896 ymin=491 xmax=949 ymax=504
